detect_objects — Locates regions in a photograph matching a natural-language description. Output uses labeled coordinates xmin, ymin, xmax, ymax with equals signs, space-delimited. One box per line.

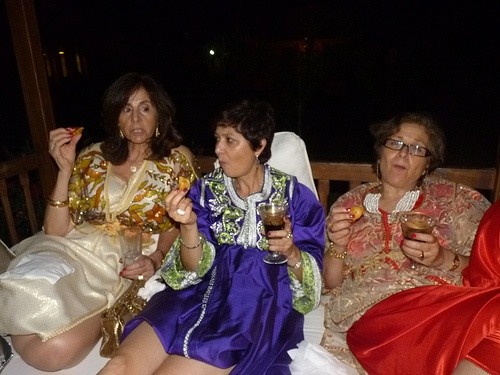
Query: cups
xmin=118 ymin=228 xmax=142 ymax=269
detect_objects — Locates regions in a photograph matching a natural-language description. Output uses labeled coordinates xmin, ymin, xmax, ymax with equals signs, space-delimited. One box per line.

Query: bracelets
xmin=157 ymin=248 xmax=164 ymax=263
xmin=47 ymin=197 xmax=69 ymax=208
xmin=329 ymin=240 xmax=346 ymax=259
xmin=287 ymin=256 xmax=303 ymax=269
xmin=179 ymin=232 xmax=203 ymax=249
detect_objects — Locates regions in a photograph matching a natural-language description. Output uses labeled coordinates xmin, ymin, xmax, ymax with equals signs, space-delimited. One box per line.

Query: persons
xmin=0 ymin=73 xmax=203 ymax=372
xmin=322 ymin=113 xmax=500 ymax=375
xmin=97 ymin=100 xmax=325 ymax=375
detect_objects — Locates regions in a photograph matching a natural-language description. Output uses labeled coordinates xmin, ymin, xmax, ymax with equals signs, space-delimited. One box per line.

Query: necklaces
xmin=127 ymin=159 xmax=142 ymax=172
xmin=364 ymin=184 xmax=420 ymax=223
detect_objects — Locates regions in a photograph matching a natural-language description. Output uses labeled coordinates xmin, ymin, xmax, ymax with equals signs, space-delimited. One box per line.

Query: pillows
xmin=215 ymin=130 xmax=319 ymax=203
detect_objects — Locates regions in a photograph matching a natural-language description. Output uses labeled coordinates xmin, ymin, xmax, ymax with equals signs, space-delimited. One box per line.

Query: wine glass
xmin=398 ymin=211 xmax=437 ymax=274
xmin=257 ymin=201 xmax=288 ymax=264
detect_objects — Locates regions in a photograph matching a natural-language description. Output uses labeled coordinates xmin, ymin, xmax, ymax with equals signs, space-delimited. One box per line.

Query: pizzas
xmin=67 ymin=127 xmax=84 ymax=136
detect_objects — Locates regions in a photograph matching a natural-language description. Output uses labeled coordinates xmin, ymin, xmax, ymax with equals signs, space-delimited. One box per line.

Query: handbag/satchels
xmin=101 ymin=295 xmax=148 ymax=358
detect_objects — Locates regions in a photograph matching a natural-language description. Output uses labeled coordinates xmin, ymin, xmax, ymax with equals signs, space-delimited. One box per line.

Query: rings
xmin=328 ymin=224 xmax=335 ymax=233
xmin=418 ymin=250 xmax=424 ymax=260
xmin=177 ymin=209 xmax=185 ymax=216
xmin=138 ymin=274 xmax=143 ymax=280
xmin=284 ymin=229 xmax=292 ymax=238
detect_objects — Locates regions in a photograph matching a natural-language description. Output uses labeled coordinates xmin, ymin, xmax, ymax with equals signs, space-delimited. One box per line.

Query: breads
xmin=350 ymin=206 xmax=363 ymax=222
xmin=179 ymin=176 xmax=190 ymax=190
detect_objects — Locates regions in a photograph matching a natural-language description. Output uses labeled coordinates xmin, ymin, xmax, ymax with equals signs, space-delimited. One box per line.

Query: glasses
xmin=382 ymin=139 xmax=431 ymax=157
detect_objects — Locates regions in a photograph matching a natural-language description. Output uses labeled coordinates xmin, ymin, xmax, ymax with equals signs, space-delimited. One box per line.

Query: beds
xmin=0 ymin=0 xmax=500 ymax=375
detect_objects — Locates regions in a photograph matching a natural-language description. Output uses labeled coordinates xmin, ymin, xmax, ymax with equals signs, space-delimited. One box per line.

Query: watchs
xmin=448 ymin=252 xmax=461 ymax=271
